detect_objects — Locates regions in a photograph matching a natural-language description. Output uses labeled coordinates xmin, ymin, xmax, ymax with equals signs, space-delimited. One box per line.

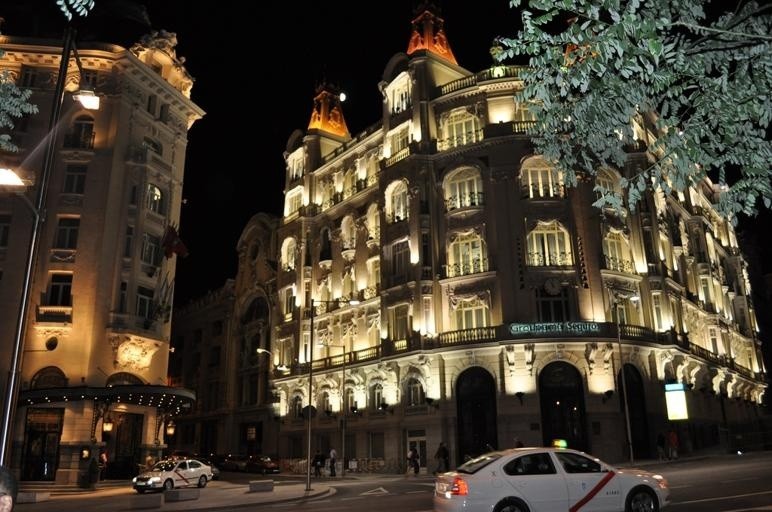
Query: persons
xmin=406 ymin=447 xmax=421 ymax=475
xmin=0 ymin=467 xmax=18 ymax=512
xmin=514 ymin=435 xmax=523 ymax=448
xmin=98 ymin=448 xmax=108 ymax=481
xmin=574 ymin=428 xmax=590 ymax=455
xmin=88 ymin=456 xmax=98 ymax=490
xmin=329 ymin=445 xmax=339 ymax=477
xmin=434 ymin=440 xmax=452 ymax=474
xmin=657 ymin=430 xmax=667 ymax=462
xmin=666 ymin=430 xmax=679 ymax=460
xmin=312 ymin=446 xmax=323 ymax=478
xmin=144 ymin=450 xmax=154 ymax=471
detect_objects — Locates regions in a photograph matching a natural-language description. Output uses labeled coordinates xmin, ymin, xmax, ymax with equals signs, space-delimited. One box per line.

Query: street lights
xmin=0 ymin=20 xmax=101 ymax=469
xmin=611 ymin=291 xmax=640 ymax=465
xmin=307 ymin=299 xmax=359 ymax=491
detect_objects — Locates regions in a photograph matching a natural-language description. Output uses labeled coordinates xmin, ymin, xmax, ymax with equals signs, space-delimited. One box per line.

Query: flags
xmin=165 ymin=227 xmax=188 ymax=259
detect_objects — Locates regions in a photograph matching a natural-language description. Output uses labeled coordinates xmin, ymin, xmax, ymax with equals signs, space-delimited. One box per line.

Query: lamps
xmin=602 ymin=391 xmax=613 ymax=404
xmin=102 ymin=417 xmax=113 ymax=432
xmin=324 ymin=410 xmax=336 ymax=418
xmin=381 ymin=403 xmax=393 ymax=415
xmin=686 ymin=383 xmax=763 ymax=409
xmin=515 ymin=392 xmax=524 ymax=406
xmin=425 ymin=398 xmax=439 ymax=410
xmin=351 ymin=407 xmax=363 ymax=417
xmin=257 ymin=347 xmax=272 ymax=356
xmin=166 ymin=421 xmax=176 ymax=435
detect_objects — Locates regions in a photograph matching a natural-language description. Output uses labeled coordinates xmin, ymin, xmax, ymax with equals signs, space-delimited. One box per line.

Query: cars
xmin=132 ymin=449 xmax=280 ymax=493
xmin=433 ymin=447 xmax=671 ymax=512
